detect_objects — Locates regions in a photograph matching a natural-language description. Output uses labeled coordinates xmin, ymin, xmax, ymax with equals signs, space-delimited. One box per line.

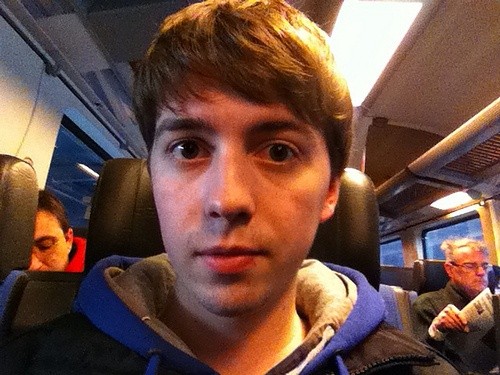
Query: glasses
xmin=452 ymin=260 xmax=492 ymax=272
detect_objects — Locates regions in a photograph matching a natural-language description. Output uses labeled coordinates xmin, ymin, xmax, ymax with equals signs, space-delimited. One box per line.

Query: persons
xmin=26 ymin=191 xmax=73 ymax=273
xmin=412 ymin=239 xmax=500 ymax=375
xmin=1 ymin=0 xmax=460 ymax=375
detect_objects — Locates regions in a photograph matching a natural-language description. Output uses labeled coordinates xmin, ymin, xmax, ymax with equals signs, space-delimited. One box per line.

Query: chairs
xmin=0 ymin=154 xmax=500 ymax=375
